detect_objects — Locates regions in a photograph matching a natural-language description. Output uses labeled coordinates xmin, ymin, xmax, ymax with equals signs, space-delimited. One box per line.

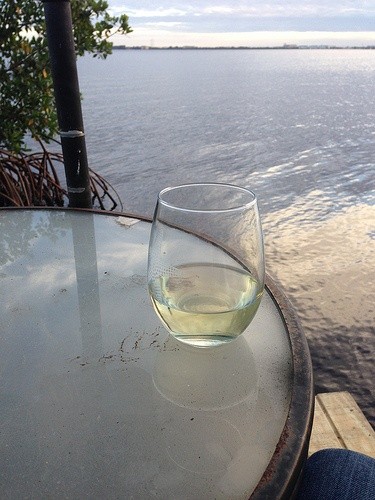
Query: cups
xmin=153 ymin=332 xmax=258 ymax=475
xmin=148 ymin=181 xmax=267 ymax=348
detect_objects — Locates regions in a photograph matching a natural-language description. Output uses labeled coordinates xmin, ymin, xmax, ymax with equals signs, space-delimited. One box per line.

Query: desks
xmin=0 ymin=206 xmax=316 ymax=500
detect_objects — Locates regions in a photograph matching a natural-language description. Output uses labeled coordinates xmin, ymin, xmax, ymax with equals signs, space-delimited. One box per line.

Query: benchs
xmin=308 ymin=391 xmax=374 ymax=460
